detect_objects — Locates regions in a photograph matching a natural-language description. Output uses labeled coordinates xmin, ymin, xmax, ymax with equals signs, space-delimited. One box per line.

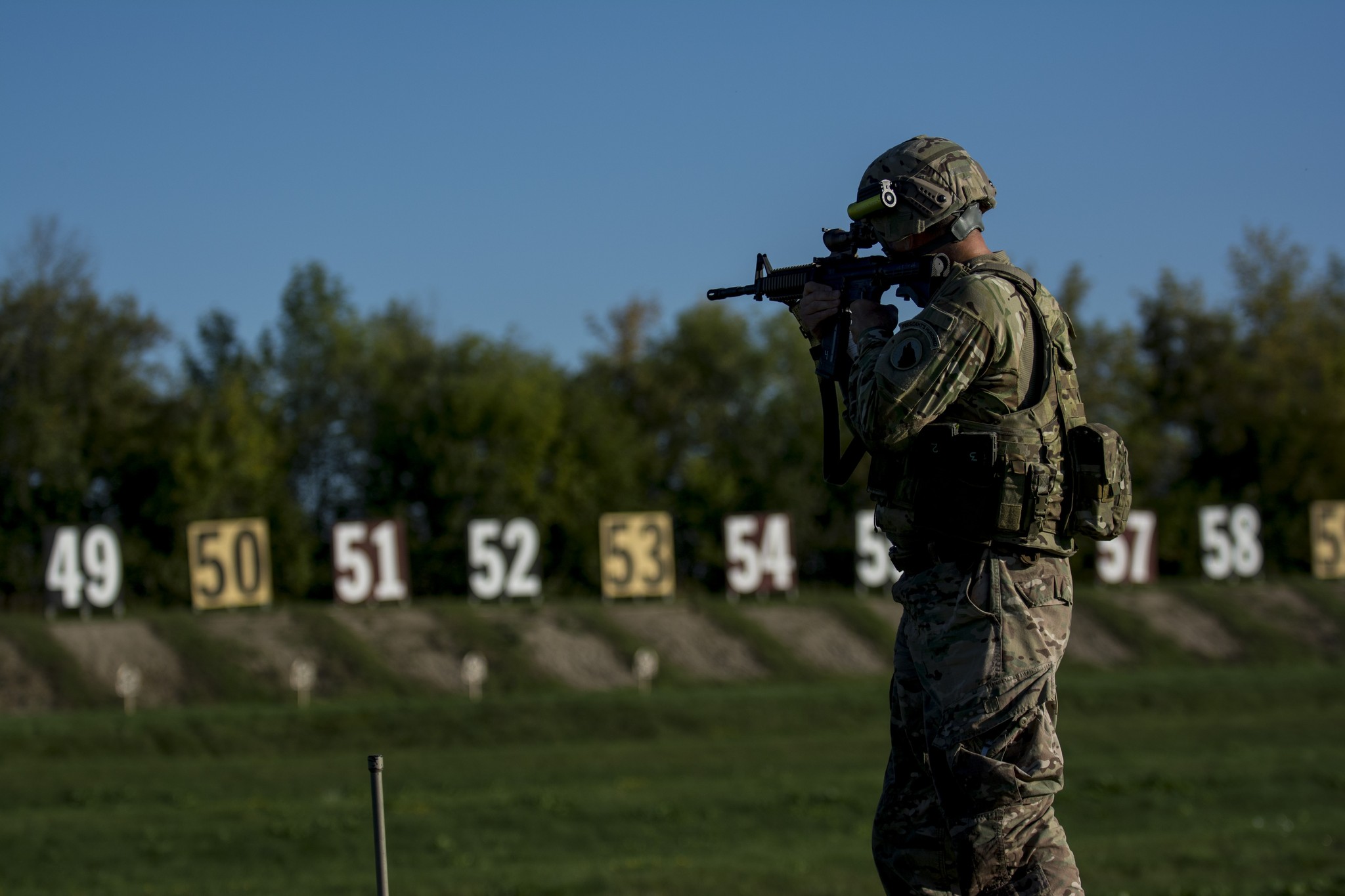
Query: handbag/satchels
xmin=1068 ymin=422 xmax=1132 ymax=543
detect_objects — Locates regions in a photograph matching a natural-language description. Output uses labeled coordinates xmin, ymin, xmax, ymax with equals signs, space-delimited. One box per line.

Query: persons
xmin=795 ymin=134 xmax=1086 ymax=895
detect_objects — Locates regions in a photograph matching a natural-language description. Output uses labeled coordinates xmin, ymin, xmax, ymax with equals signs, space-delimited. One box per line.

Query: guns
xmin=705 ymin=219 xmax=950 ymax=384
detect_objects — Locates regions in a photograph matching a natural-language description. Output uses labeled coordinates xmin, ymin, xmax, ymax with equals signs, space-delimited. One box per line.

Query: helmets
xmin=857 ymin=134 xmax=997 ymax=249
